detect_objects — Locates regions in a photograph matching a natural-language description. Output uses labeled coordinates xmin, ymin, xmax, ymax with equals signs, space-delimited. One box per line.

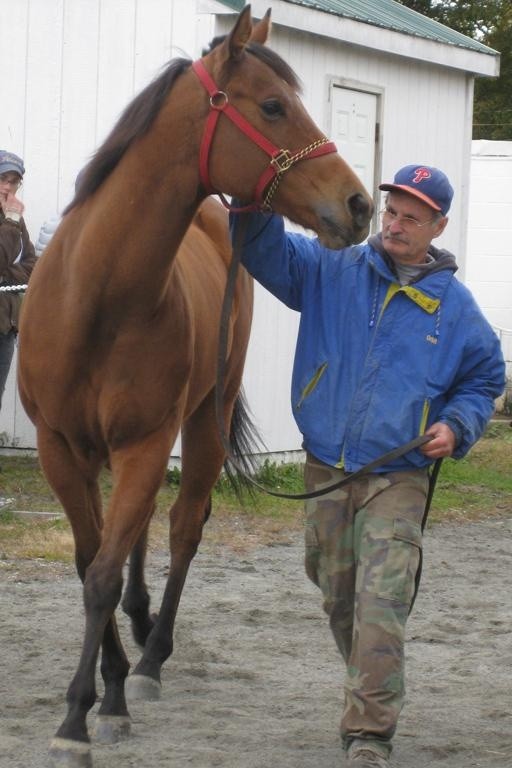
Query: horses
xmin=14 ymin=2 xmax=378 ymax=768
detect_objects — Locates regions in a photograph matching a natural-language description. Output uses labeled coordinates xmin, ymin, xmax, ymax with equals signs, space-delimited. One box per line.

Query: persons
xmin=0 ymin=146 xmax=39 ymax=404
xmin=226 ymin=163 xmax=506 ymax=767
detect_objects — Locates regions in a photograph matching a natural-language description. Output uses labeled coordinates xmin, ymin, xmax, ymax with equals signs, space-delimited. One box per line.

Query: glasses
xmin=376 ymin=202 xmax=440 ymax=234
xmin=1 ymin=174 xmax=23 ymax=189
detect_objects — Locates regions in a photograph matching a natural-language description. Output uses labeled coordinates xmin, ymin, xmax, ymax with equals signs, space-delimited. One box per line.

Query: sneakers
xmin=342 ymin=734 xmax=395 ymax=768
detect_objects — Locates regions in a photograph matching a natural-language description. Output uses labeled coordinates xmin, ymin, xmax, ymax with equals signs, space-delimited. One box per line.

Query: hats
xmin=1 ymin=147 xmax=28 ymax=181
xmin=377 ymin=161 xmax=455 ymax=217
